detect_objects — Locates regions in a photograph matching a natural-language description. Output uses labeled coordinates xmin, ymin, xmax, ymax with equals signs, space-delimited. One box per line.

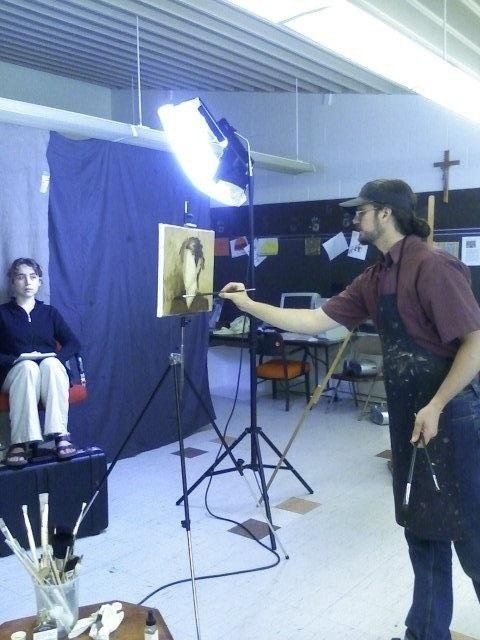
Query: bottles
xmin=338 ymin=179 xmax=417 ymax=209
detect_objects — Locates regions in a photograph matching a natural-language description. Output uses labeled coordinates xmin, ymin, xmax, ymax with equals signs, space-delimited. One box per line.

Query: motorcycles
xmin=176 ymin=174 xmax=313 ymax=551
xmin=66 ymin=319 xmax=290 ymax=640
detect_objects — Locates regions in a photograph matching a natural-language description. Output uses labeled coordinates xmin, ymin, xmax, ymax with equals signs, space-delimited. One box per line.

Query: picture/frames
xmin=207 ymin=325 xmax=354 ymax=405
xmin=0 ymin=600 xmax=169 ymax=640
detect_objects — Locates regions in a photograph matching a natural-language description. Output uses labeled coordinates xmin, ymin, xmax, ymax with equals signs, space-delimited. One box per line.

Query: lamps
xmin=324 ymin=324 xmax=384 ymax=420
xmin=253 ymin=331 xmax=314 ymax=412
xmin=2 ymin=341 xmax=90 ymax=452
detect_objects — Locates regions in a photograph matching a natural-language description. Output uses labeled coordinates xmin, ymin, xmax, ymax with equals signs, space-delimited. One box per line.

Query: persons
xmin=170 ymin=237 xmax=211 ymax=315
xmin=0 ymin=258 xmax=83 ymax=466
xmin=217 ymin=180 xmax=480 ymax=640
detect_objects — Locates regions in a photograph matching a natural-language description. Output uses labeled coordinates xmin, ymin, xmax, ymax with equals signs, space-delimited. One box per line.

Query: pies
xmin=175 ymin=288 xmax=256 ymax=300
xmin=420 ymin=432 xmax=442 ymax=495
xmin=0 ymin=492 xmax=87 ymax=624
xmin=399 ymin=440 xmax=419 ymax=515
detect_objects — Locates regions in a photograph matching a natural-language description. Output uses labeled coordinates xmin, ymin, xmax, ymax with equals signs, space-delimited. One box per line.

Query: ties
xmin=280 ymin=293 xmax=321 ymax=310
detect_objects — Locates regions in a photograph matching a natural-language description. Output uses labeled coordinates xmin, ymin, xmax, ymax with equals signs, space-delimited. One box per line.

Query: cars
xmin=35 ymin=574 xmax=80 ymax=637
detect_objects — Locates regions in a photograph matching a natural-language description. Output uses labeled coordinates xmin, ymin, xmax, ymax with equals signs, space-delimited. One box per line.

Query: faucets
xmin=6 ymin=443 xmax=28 ymax=467
xmin=54 ymin=435 xmax=77 ymax=459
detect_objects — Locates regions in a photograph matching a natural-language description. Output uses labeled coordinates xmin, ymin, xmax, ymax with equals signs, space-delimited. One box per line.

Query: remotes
xmin=308 ymin=338 xmax=318 ymax=341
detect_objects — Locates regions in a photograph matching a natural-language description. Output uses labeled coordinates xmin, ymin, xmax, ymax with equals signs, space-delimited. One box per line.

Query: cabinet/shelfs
xmin=141 ymin=609 xmax=160 ymax=640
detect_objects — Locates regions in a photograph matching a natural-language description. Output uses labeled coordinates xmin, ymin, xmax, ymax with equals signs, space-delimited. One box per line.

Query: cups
xmin=353 ymin=206 xmax=378 ymax=219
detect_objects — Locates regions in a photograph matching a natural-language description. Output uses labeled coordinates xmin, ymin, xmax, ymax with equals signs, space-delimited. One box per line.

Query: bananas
xmin=277 ymin=333 xmax=312 ymax=340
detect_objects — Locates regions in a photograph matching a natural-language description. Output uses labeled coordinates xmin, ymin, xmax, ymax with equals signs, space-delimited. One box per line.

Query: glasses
xmin=155 ymin=99 xmax=317 ymax=550
